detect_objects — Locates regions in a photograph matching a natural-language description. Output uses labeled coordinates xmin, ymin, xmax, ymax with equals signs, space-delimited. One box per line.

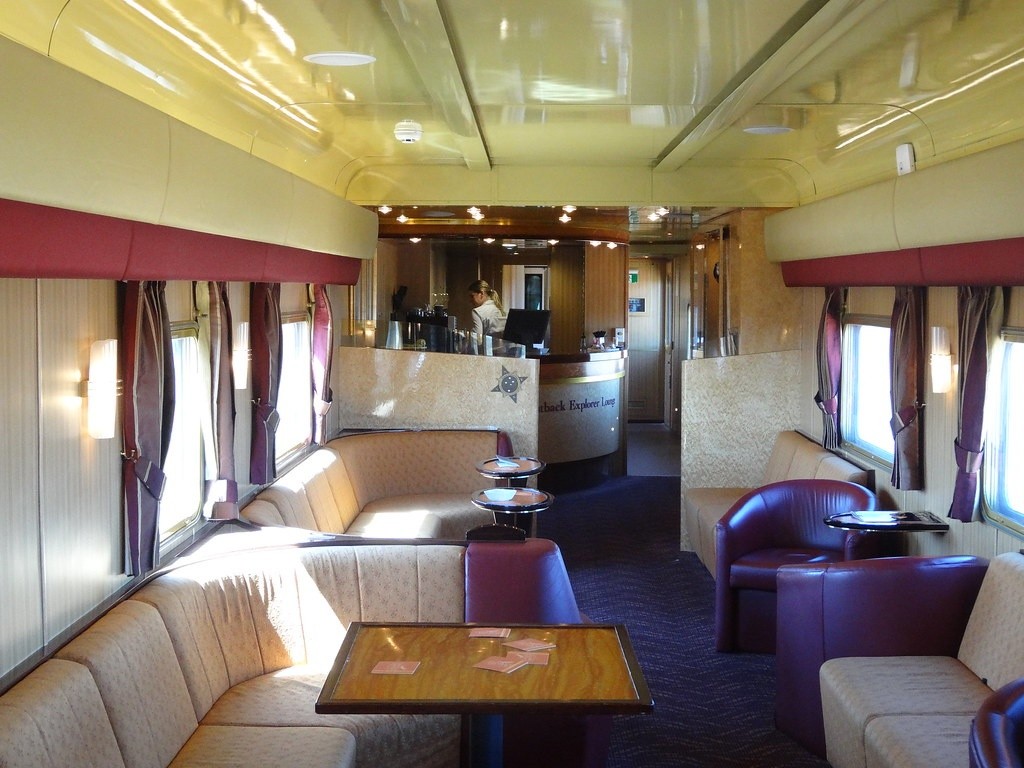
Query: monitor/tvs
xmin=503 ymin=308 xmax=552 ymax=356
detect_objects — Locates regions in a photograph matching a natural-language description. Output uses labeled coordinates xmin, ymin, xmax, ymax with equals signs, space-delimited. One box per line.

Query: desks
xmin=469 ymin=487 xmax=555 ymax=525
xmin=475 ymin=456 xmax=547 ymax=487
xmin=315 ymin=620 xmax=657 ymax=713
xmin=823 ymin=509 xmax=949 ymax=533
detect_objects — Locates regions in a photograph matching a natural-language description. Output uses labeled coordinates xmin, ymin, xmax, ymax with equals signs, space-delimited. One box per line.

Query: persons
xmin=468 ymin=280 xmax=507 ymax=346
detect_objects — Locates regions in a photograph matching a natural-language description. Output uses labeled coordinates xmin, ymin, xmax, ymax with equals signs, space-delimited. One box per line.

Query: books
xmin=850 ymin=511 xmax=929 ymax=524
xmin=495 ymin=454 xmax=520 ymax=468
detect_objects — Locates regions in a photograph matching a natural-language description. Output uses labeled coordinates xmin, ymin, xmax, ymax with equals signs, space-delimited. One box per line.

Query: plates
xmin=403 ymin=345 xmax=428 ymax=350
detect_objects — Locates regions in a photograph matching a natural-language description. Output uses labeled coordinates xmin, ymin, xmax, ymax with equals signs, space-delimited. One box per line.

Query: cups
xmin=596 ymin=337 xmax=605 ymax=345
xmin=612 ymin=337 xmax=618 ymax=347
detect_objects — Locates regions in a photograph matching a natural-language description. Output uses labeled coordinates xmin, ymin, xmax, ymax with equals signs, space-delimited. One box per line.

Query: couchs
xmin=1 ymin=427 xmax=613 ymax=768
xmin=683 ymin=428 xmax=1024 ymax=768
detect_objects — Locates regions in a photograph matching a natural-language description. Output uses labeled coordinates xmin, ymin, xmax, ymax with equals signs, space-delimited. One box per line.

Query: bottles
xmin=580 ymin=332 xmax=587 ymax=347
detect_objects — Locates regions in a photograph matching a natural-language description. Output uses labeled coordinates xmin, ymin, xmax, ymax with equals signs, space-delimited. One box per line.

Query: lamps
xmin=929 ymin=326 xmax=955 ymax=395
